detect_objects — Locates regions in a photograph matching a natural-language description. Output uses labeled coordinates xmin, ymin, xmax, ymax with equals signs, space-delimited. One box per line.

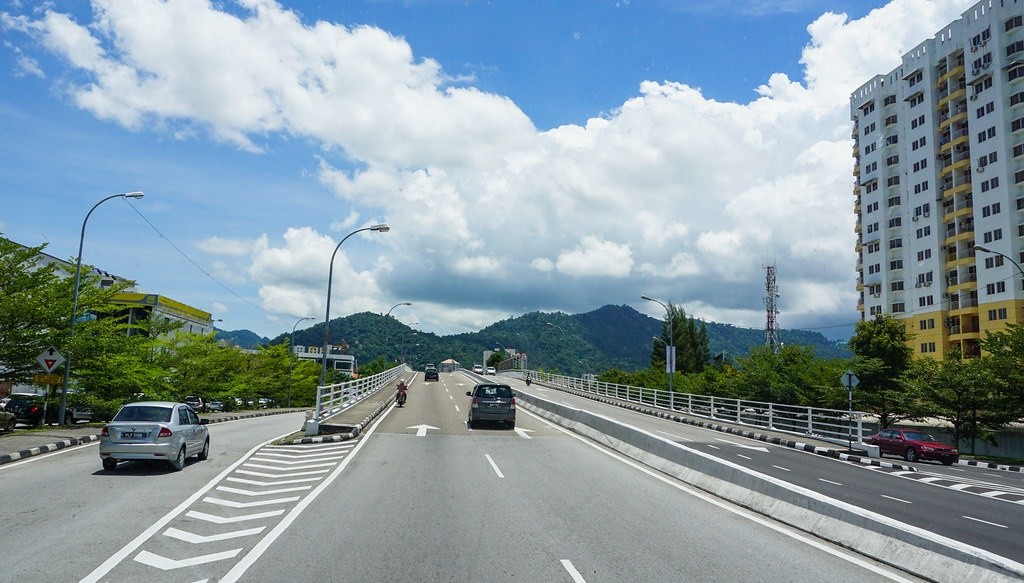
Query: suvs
xmin=183 ymin=396 xmax=204 ymax=413
xmin=0 ymin=392 xmax=109 ymax=432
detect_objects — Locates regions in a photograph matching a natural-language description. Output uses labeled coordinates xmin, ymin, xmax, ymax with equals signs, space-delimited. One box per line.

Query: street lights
xmin=578 ymin=360 xmax=590 ymax=372
xmin=319 ymin=224 xmax=390 ymax=412
xmin=288 ymin=317 xmax=315 ymax=408
xmin=497 ymin=342 xmax=506 ymax=353
xmin=516 ymin=332 xmax=530 ymax=346
xmin=547 ymin=322 xmax=565 ymax=356
xmin=385 ymin=303 xmax=422 ymax=369
xmin=58 ymin=192 xmax=145 ymax=426
xmin=640 ymin=295 xmax=672 ymax=412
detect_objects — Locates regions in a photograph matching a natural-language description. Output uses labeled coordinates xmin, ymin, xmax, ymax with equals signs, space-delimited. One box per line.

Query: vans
xmin=468 ymin=380 xmax=516 ymax=430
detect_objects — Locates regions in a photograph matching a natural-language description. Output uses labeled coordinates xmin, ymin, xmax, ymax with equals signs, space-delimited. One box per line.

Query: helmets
xmin=400 ymin=379 xmax=404 ymax=381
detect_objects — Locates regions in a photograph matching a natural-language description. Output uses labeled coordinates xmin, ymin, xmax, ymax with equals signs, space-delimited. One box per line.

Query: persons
xmin=394 ymin=379 xmax=408 ymax=403
xmin=526 ymin=372 xmax=532 ymax=384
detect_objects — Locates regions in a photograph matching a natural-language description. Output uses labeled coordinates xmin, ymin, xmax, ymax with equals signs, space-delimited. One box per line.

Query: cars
xmin=130 ymin=393 xmax=146 ymax=400
xmin=486 ymin=366 xmax=497 ymax=376
xmin=424 ymin=363 xmax=439 ymax=381
xmin=866 ymin=428 xmax=959 ymax=466
xmin=100 ymin=402 xmax=210 ymax=471
xmin=208 ymin=397 xmax=282 ymax=412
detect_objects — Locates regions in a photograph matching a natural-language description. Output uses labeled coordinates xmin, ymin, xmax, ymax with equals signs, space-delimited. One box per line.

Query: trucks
xmin=472 ymin=365 xmax=484 ymax=374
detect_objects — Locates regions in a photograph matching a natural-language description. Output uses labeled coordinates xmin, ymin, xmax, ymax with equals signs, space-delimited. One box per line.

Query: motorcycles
xmin=525 ymin=376 xmax=532 ymax=386
xmin=395 ymin=385 xmax=408 ymax=407
